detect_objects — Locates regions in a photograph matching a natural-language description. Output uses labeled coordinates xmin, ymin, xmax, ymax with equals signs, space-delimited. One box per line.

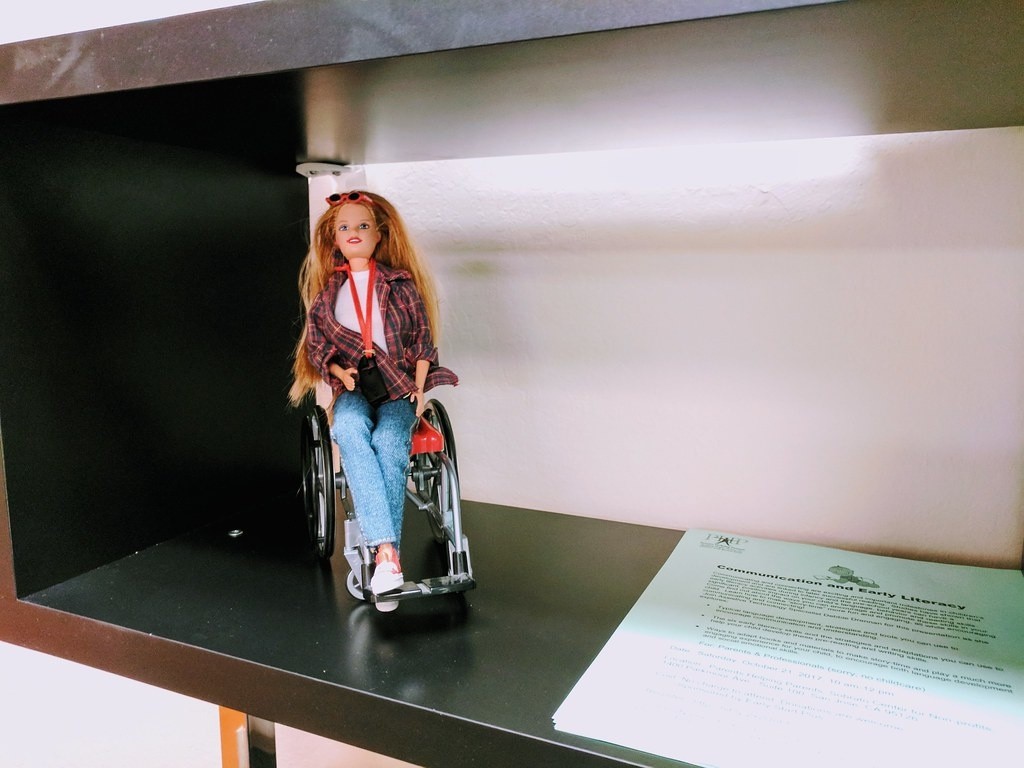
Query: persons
xmin=288 ymin=191 xmax=459 ymax=612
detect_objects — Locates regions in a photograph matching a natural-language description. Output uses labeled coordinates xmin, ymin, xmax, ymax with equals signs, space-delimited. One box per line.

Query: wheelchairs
xmin=298 ymin=399 xmax=478 ymax=604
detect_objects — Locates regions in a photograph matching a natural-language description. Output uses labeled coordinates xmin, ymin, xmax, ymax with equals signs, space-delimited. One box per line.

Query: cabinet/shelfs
xmin=1 ymin=0 xmax=1021 ymax=768
